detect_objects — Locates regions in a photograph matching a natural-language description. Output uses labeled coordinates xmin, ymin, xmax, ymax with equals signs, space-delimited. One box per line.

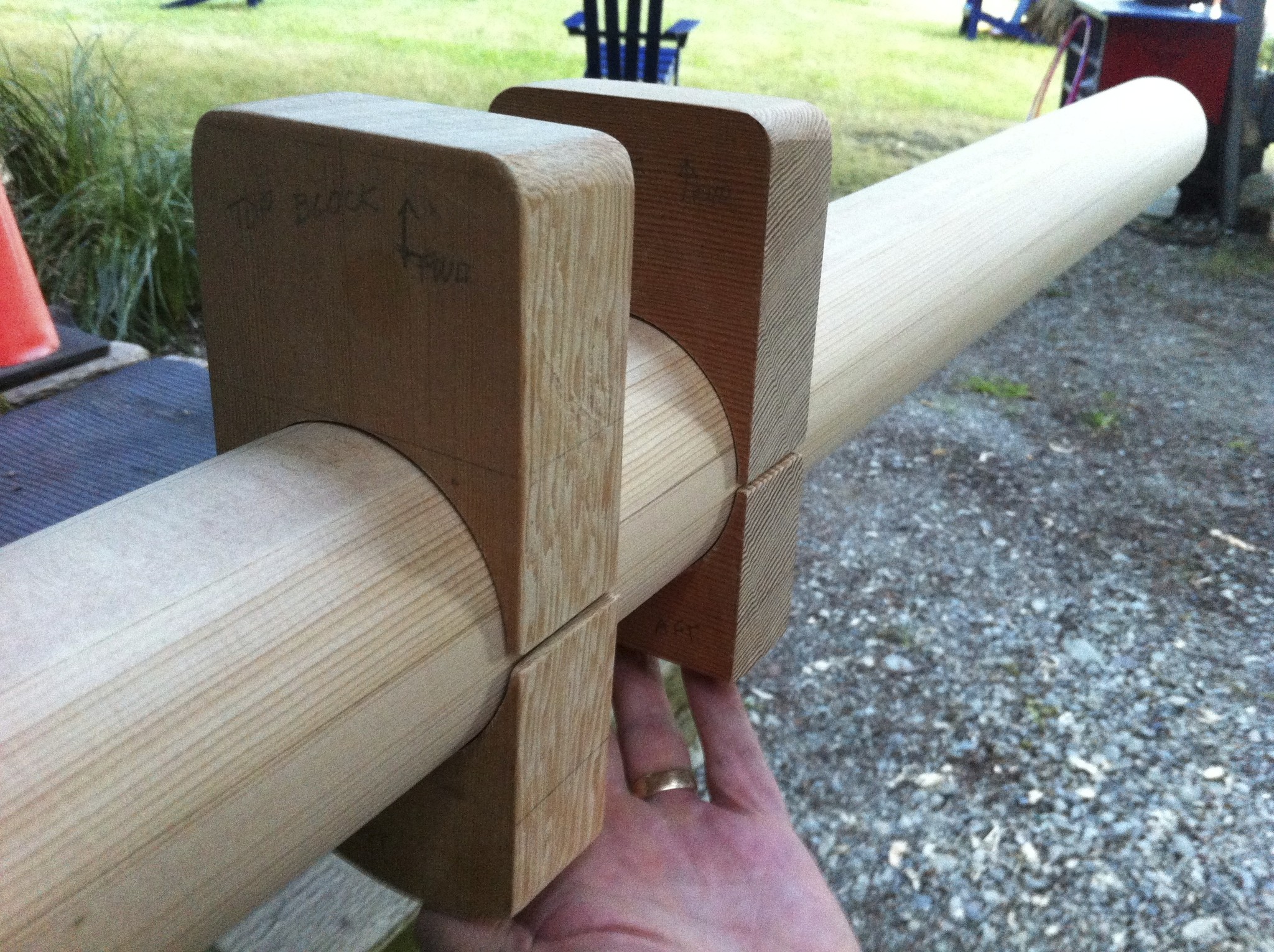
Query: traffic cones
xmin=0 ymin=170 xmax=110 ymax=392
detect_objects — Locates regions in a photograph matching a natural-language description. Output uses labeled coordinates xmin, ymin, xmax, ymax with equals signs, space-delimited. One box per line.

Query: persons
xmin=415 ymin=646 xmax=867 ymax=952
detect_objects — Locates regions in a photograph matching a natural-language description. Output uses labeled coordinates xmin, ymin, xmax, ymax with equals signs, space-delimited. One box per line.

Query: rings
xmin=627 ymin=765 xmax=698 ymax=800
